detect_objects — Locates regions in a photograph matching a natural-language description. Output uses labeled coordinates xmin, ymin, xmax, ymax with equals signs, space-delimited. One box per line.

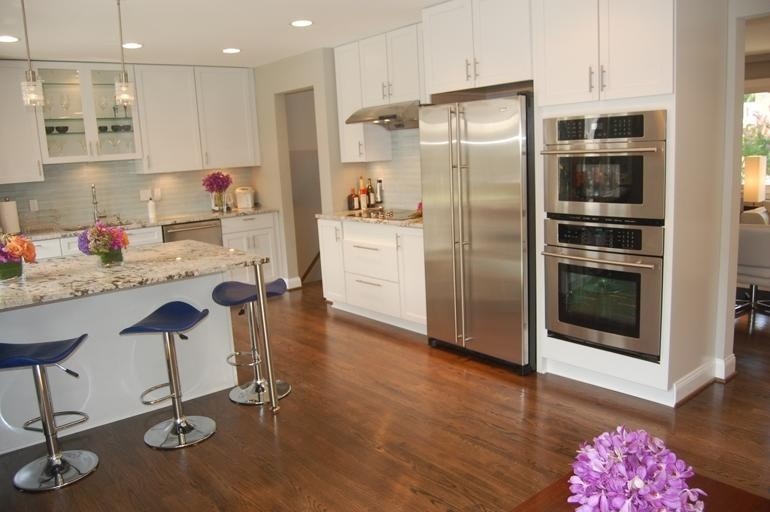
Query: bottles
xmin=148 ymin=197 xmax=157 ymax=222
xmin=348 ymin=176 xmax=384 ymax=212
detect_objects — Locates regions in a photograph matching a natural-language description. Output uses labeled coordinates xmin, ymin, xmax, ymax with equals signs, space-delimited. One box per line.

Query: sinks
xmin=58 ymin=223 xmax=145 ymax=231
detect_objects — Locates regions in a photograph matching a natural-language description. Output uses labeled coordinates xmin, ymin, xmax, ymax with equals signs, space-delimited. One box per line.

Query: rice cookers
xmin=236 ymin=186 xmax=255 ymax=209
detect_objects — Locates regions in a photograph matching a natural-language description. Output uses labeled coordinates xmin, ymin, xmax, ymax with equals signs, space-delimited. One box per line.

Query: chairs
xmin=736 ymin=207 xmax=770 ymax=335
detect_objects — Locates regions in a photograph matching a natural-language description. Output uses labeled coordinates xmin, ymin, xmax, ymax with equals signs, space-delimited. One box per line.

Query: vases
xmin=1 ymin=257 xmax=22 ymax=282
xmin=210 ymin=190 xmax=227 ymax=212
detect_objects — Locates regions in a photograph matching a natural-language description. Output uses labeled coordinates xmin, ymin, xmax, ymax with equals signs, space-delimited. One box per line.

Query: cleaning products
xmin=148 ymin=197 xmax=157 ymax=227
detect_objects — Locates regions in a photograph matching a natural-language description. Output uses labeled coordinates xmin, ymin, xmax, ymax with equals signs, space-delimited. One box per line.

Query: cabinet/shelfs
xmin=333 ymin=39 xmax=394 ymax=163
xmin=1 ymin=62 xmax=262 ymax=183
xmin=14 ymin=212 xmax=282 ymax=282
xmin=342 ymin=222 xmax=400 ymax=321
xmin=396 ymin=228 xmax=426 ymax=325
xmin=316 ymin=218 xmax=345 ymax=299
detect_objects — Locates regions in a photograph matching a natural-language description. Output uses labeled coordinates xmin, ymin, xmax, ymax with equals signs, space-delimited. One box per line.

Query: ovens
xmin=541 ymin=109 xmax=666 ymax=366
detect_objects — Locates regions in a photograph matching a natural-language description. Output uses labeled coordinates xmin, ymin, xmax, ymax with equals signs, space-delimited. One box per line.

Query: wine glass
xmin=41 ymin=93 xmax=71 ymax=114
xmin=96 ymin=95 xmax=129 ymax=117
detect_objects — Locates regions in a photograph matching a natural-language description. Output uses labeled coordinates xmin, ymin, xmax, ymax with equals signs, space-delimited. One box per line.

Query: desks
xmin=506 ymin=467 xmax=768 ymax=510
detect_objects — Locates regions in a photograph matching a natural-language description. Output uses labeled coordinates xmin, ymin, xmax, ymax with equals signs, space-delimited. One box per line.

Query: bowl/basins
xmin=96 ymin=124 xmax=131 ymax=131
xmin=44 ymin=125 xmax=69 ymax=133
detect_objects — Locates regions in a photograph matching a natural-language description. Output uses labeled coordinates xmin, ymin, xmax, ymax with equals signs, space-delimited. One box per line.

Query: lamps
xmin=20 ymin=1 xmax=45 ymax=108
xmin=743 ymin=154 xmax=766 ymax=208
xmin=114 ymin=1 xmax=137 ymax=106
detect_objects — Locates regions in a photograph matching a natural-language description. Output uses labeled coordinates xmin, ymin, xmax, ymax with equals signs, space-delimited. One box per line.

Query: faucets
xmin=92 ymin=183 xmax=100 ymax=224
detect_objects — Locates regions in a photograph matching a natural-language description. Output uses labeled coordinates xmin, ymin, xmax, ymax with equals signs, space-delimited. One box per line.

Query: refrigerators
xmin=418 ymin=88 xmax=538 ymax=377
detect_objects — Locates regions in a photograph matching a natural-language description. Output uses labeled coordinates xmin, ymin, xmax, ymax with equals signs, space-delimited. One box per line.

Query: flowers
xmin=0 ymin=232 xmax=35 ymax=265
xmin=567 ymin=426 xmax=707 ymax=510
xmin=204 ymin=172 xmax=231 ymax=207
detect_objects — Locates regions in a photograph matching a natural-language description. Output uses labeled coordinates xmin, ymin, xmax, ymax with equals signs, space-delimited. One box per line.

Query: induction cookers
xmin=347 ymin=206 xmax=419 ymax=221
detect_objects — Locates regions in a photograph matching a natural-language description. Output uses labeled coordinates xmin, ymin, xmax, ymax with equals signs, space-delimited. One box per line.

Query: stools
xmin=1 ymin=331 xmax=101 ymax=494
xmin=211 ymin=278 xmax=293 ymax=408
xmin=119 ymin=300 xmax=217 ymax=452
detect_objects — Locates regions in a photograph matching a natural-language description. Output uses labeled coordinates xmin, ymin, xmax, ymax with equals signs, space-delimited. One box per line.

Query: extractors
xmin=346 ymin=100 xmax=419 ymax=131
